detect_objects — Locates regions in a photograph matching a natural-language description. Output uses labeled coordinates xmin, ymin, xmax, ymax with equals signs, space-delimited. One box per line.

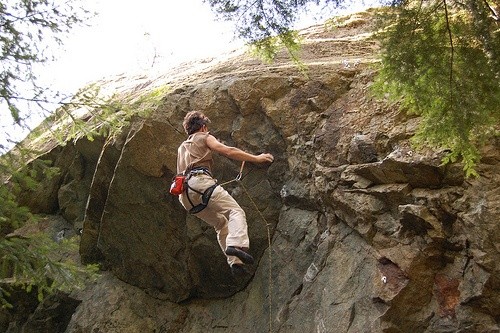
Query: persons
xmin=175 ymin=110 xmax=274 ymax=283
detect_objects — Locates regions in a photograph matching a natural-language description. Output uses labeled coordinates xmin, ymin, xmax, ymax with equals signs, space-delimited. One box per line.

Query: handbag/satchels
xmin=170 ymin=176 xmax=185 ymax=196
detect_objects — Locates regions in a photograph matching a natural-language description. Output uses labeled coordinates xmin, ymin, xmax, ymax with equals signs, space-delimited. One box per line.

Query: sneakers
xmin=226 ymin=246 xmax=254 ymax=264
xmin=232 ymin=264 xmax=252 ymax=281
